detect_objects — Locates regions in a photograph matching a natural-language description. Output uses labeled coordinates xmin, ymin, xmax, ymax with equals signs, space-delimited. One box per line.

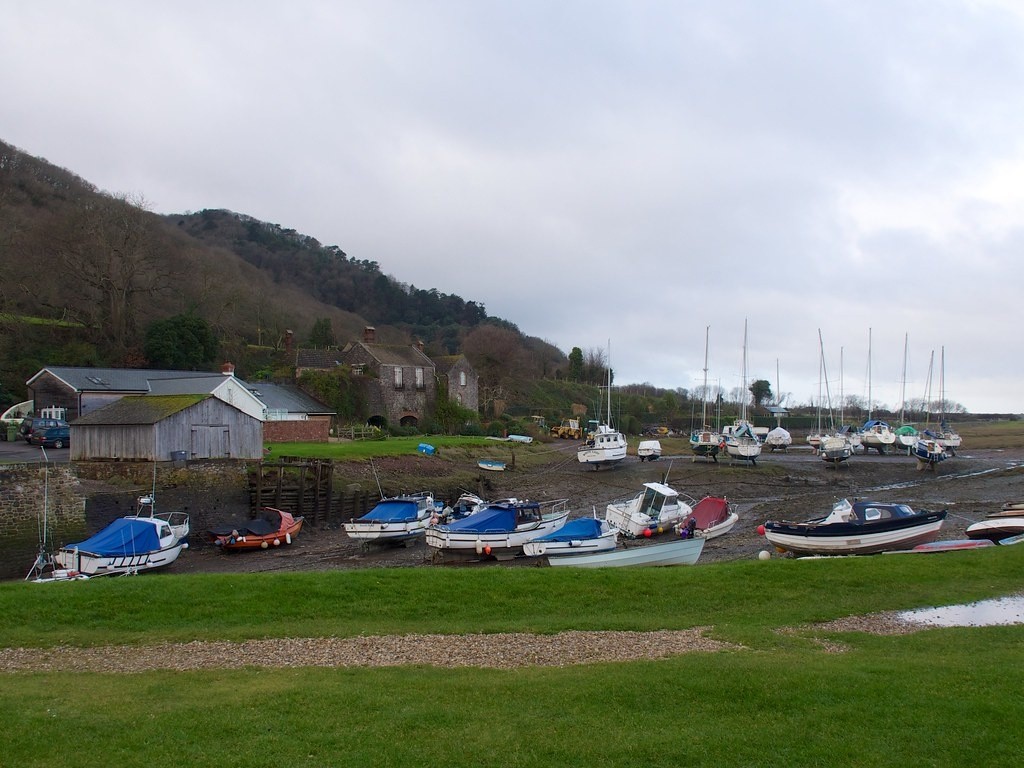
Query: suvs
xmin=19 ymin=417 xmax=70 ymax=444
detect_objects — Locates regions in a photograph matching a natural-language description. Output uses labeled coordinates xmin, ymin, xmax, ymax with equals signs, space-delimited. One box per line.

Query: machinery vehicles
xmin=551 ymin=417 xmax=583 ymax=440
xmin=529 ymin=416 xmax=550 ymax=439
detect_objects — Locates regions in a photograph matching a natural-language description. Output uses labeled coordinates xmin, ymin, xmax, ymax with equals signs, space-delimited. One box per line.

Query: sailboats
xmin=927 ymin=346 xmax=963 ymax=456
xmin=831 ymin=345 xmax=861 ymax=449
xmin=727 ymin=317 xmax=762 ymax=467
xmin=809 ymin=349 xmax=832 ymax=456
xmin=763 ymin=357 xmax=793 ymax=455
xmin=912 ymin=348 xmax=948 ymax=473
xmin=858 ymin=327 xmax=895 ymax=456
xmin=692 ymin=325 xmax=723 ymax=464
xmin=576 ymin=339 xmax=628 ymax=468
xmin=891 ymin=333 xmax=924 ymax=458
xmin=816 ymin=328 xmax=854 ymax=470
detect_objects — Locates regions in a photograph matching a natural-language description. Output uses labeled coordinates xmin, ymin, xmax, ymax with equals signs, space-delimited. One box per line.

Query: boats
xmin=208 ymin=506 xmax=304 ymax=554
xmin=424 ymin=497 xmax=569 ymax=564
xmin=25 ymin=445 xmax=191 ymax=585
xmin=638 ymin=440 xmax=662 ymax=462
xmin=520 ymin=518 xmax=621 ymax=558
xmin=761 ymin=501 xmax=947 ymax=558
xmin=476 ymin=461 xmax=508 ymax=472
xmin=672 ymin=495 xmax=738 ymax=543
xmin=882 ymin=540 xmax=999 ymax=555
xmin=604 ymin=457 xmax=694 ymax=538
xmin=343 ymin=457 xmax=443 ymax=549
xmin=965 ymin=502 xmax=1024 ymax=538
xmin=549 ymin=534 xmax=705 ymax=568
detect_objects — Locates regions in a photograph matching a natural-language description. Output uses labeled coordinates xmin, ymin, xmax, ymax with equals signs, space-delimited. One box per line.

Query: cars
xmin=32 ymin=426 xmax=70 ymax=449
